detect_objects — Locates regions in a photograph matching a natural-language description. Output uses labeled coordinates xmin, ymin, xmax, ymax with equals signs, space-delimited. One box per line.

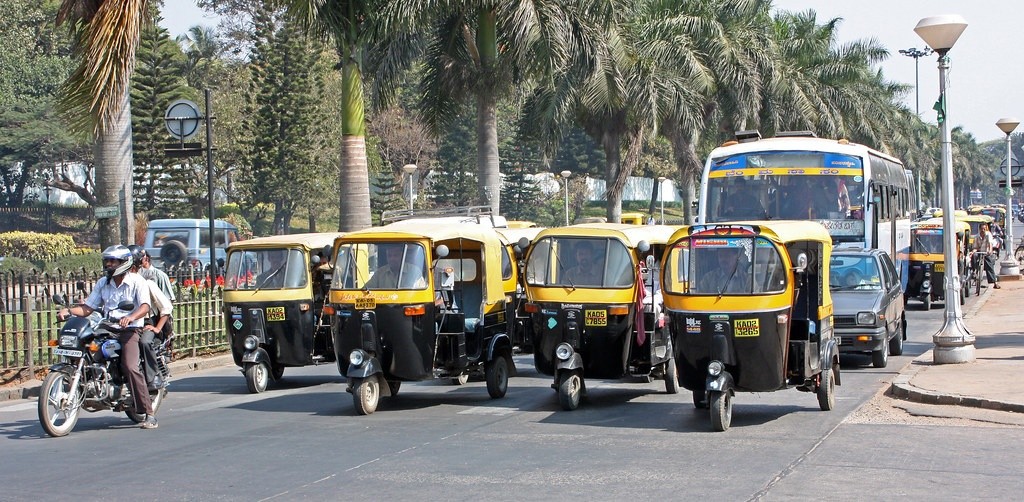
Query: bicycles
xmin=971 ymin=251 xmax=988 ymax=296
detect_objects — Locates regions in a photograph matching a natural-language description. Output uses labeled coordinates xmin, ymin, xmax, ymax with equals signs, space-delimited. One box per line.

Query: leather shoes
xmin=148 ymin=374 xmax=164 ymax=391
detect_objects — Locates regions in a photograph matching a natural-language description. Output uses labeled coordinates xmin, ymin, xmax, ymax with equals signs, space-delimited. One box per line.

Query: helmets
xmin=101 ymin=245 xmax=133 ymax=277
xmin=129 ymin=245 xmax=146 ymax=266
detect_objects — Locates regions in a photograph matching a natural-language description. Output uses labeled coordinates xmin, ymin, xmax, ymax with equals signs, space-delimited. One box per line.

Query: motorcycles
xmin=910 ymin=205 xmax=1009 ymax=311
xmin=323 ymin=219 xmax=517 ymax=416
xmin=217 ymin=232 xmax=349 ymax=393
xmin=38 ymin=287 xmax=175 ymax=437
xmin=518 ymin=222 xmax=690 ymax=410
xmin=646 ymin=220 xmax=841 ymax=432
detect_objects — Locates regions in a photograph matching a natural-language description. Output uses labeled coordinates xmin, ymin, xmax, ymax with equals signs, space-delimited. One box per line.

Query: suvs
xmin=143 ymin=219 xmax=259 ymax=288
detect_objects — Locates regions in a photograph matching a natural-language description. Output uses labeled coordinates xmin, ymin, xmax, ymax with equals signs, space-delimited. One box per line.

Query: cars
xmin=829 ymin=245 xmax=907 ymax=367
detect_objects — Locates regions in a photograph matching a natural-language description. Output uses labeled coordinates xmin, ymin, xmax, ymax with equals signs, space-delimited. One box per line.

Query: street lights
xmin=561 ymin=170 xmax=571 ymax=226
xmin=42 ymin=186 xmax=52 ymax=233
xmin=914 ymin=16 xmax=976 ymax=365
xmin=996 ymin=118 xmax=1022 ymax=281
xmin=658 ymin=177 xmax=666 ymax=224
xmin=898 ymin=46 xmax=934 ymax=211
xmin=403 ymin=164 xmax=417 ymax=215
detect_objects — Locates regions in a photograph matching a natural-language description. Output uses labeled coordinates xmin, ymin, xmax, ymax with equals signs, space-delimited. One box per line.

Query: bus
xmin=695 ymin=128 xmax=915 ymax=304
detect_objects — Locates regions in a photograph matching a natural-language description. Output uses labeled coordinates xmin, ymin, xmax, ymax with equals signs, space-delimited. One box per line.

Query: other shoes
xmin=141 ymin=416 xmax=158 ymax=429
xmin=972 ymin=272 xmax=977 ymax=279
xmin=993 ymin=284 xmax=1001 ymax=290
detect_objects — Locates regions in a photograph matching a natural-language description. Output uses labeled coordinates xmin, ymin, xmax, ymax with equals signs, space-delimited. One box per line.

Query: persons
xmin=966 ymin=223 xmax=1003 ymax=288
xmin=255 ymin=250 xmax=317 ymax=325
xmin=312 ymin=251 xmax=342 ymax=289
xmin=56 ymin=244 xmax=175 ymax=429
xmin=699 ymin=249 xmax=759 ymax=291
xmin=721 ymin=177 xmax=767 ymax=219
xmin=362 ymin=245 xmax=424 ymax=289
xmin=562 ymin=241 xmax=613 ymax=285
xmin=844 ymin=271 xmax=872 ymax=289
